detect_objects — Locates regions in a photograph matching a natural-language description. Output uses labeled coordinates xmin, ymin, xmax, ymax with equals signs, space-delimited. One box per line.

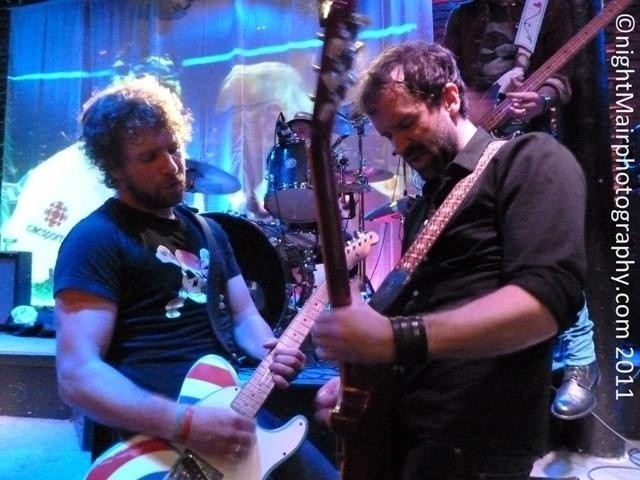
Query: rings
xmin=523 ymin=110 xmax=528 ymax=120
xmin=232 ymin=444 xmax=242 ymax=456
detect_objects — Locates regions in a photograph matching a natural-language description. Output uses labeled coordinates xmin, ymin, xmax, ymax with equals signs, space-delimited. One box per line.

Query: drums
xmin=194 ymin=211 xmax=325 ymax=362
xmin=263 ymin=139 xmax=341 ymax=222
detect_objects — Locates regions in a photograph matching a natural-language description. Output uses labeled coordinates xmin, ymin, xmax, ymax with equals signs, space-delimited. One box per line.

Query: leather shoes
xmin=551 ymin=360 xmax=601 ymax=421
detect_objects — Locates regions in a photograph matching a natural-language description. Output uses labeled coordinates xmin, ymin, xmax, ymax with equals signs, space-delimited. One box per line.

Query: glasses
xmin=113 ymin=59 xmax=151 ymax=74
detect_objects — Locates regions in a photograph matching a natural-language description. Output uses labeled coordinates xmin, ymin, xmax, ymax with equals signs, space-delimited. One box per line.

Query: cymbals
xmin=333 ymin=101 xmax=378 ymax=135
xmin=332 ymin=168 xmax=394 ymax=192
xmin=184 ymin=158 xmax=240 ymax=195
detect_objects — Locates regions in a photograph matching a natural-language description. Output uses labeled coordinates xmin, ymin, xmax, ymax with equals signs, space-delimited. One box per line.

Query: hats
xmin=287 ymin=111 xmax=314 ymax=130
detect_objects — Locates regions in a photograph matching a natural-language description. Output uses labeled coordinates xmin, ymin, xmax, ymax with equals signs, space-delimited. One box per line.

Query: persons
xmin=51 ymin=72 xmax=342 ymax=479
xmin=311 ymin=41 xmax=589 ymax=480
xmin=247 ymin=110 xmax=313 ymax=218
xmin=443 ymin=0 xmax=602 ymax=421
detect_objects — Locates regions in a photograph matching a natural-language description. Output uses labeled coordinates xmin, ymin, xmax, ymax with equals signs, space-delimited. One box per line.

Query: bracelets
xmin=539 ymin=92 xmax=553 ymax=119
xmin=173 ymin=403 xmax=193 ymax=446
xmin=388 ymin=313 xmax=429 ymax=375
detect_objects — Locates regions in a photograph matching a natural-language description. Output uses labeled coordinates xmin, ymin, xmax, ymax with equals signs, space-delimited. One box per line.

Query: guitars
xmin=311 ymin=1 xmax=389 ymax=480
xmin=84 ymin=229 xmax=379 ymax=480
xmin=465 ymin=0 xmax=633 ymax=143
